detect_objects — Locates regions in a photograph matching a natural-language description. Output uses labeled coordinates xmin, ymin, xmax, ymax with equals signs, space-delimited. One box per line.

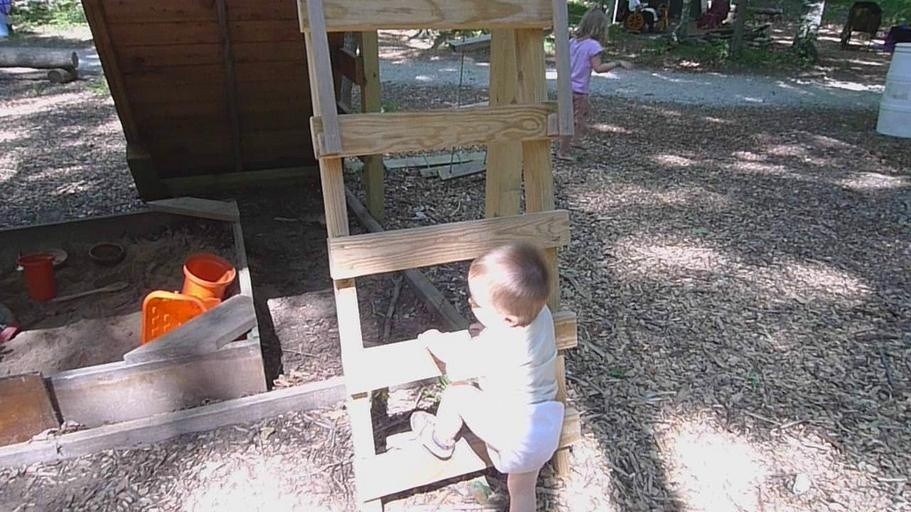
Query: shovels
xmin=46 ymin=280 xmax=129 ymax=302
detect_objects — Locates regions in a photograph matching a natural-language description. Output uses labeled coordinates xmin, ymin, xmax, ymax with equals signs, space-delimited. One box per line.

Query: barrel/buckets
xmin=16 ymin=255 xmax=54 ymax=300
xmin=179 ymin=253 xmax=236 ymax=302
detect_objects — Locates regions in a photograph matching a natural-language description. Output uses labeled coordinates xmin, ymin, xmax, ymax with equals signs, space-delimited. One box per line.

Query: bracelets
xmin=614 ymin=60 xmax=622 ymax=68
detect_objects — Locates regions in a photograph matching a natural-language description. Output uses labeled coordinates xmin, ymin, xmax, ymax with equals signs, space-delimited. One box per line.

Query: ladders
xmin=298 ymin=0 xmax=576 ymax=512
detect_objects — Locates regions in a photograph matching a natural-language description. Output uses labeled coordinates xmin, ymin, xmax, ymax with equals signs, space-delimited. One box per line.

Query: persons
xmin=801 ymin=0 xmax=826 ymax=39
xmin=555 ymin=11 xmax=636 ymax=161
xmin=406 ymin=239 xmax=568 ymax=512
xmin=630 ymin=0 xmax=660 ymax=34
